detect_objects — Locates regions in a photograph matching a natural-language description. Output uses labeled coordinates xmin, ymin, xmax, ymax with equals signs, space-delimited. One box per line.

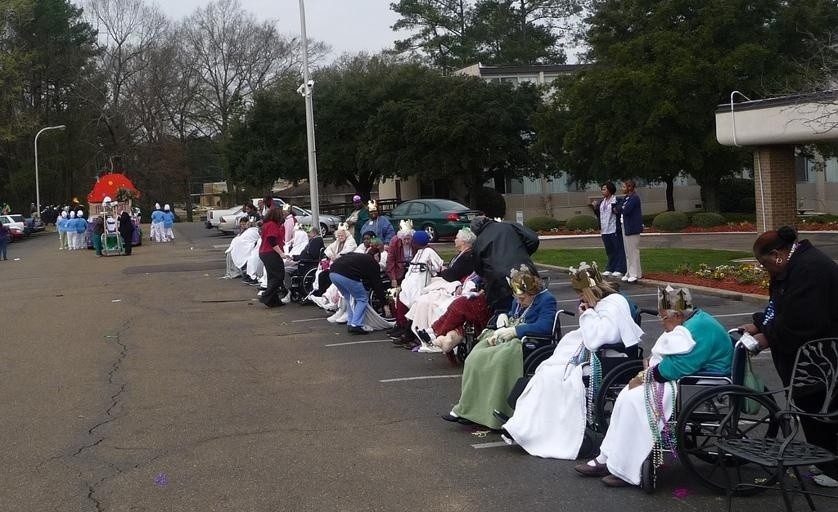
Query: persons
xmin=31 ymin=196 xmax=134 ymax=257
xmin=149 ymin=202 xmax=175 ymax=243
xmin=0 ymin=203 xmax=13 ymax=261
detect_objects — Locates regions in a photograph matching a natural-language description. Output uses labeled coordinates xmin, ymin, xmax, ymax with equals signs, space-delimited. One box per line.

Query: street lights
xmin=34 ymin=124 xmax=68 ymax=220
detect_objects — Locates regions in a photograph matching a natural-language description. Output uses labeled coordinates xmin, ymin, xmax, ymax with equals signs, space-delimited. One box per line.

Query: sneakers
xmin=601 ymin=270 xmax=637 ymax=283
xmin=808 ymin=464 xmax=823 ymax=475
xmin=387 ymin=325 xmax=443 ymax=352
xmin=241 ymin=276 xmax=260 ymax=286
xmin=310 ymin=295 xmax=348 ymax=323
xmin=813 ymin=473 xmax=838 ymax=486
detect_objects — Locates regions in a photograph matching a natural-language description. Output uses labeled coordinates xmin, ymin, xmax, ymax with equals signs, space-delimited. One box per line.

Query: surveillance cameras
xmin=297 ymin=83 xmax=304 ymax=93
xmin=307 ymin=80 xmax=314 ymax=89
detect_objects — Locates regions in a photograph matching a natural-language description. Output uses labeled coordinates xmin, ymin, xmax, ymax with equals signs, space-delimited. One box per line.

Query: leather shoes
xmin=348 ymin=325 xmax=369 ymax=334
xmin=441 ymin=412 xmax=459 ymax=422
xmin=603 ymin=472 xmax=627 ymax=487
xmin=264 ymin=302 xmax=273 ymax=307
xmin=573 ymin=457 xmax=607 ymax=477
xmin=458 ymin=414 xmax=471 ymax=425
xmin=278 ymin=300 xmax=286 ymax=306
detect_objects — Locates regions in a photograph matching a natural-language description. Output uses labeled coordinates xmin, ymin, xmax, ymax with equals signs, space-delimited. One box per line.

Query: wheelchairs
xmin=288 ymin=246 xmax=487 ymax=364
xmin=475 ymin=308 xmax=576 ymax=379
xmin=638 ymin=323 xmax=783 ymax=498
xmin=493 ymin=307 xmax=664 ymax=440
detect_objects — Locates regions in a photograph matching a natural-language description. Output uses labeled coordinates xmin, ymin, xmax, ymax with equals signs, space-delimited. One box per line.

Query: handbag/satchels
xmin=739 ymin=347 xmax=765 ymax=416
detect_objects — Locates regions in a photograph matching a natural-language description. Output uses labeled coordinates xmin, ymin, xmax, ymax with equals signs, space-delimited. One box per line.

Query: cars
xmin=0 ymin=214 xmax=46 ymax=243
xmin=382 ymin=197 xmax=488 ymax=244
xmin=205 ymin=197 xmax=342 ymax=240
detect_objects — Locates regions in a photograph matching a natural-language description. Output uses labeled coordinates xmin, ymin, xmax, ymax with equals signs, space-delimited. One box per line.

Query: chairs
xmin=707 ymin=336 xmax=838 ymax=512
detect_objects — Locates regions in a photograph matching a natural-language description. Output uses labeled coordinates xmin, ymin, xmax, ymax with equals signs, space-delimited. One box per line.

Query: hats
xmin=61 ymin=210 xmax=67 ymax=219
xmin=70 ymin=211 xmax=76 ymax=218
xmin=77 ymin=210 xmax=84 ymax=218
xmin=155 ymin=203 xmax=161 ymax=209
xmin=413 ymin=231 xmax=429 ymax=246
xmin=164 ymin=204 xmax=170 ymax=210
